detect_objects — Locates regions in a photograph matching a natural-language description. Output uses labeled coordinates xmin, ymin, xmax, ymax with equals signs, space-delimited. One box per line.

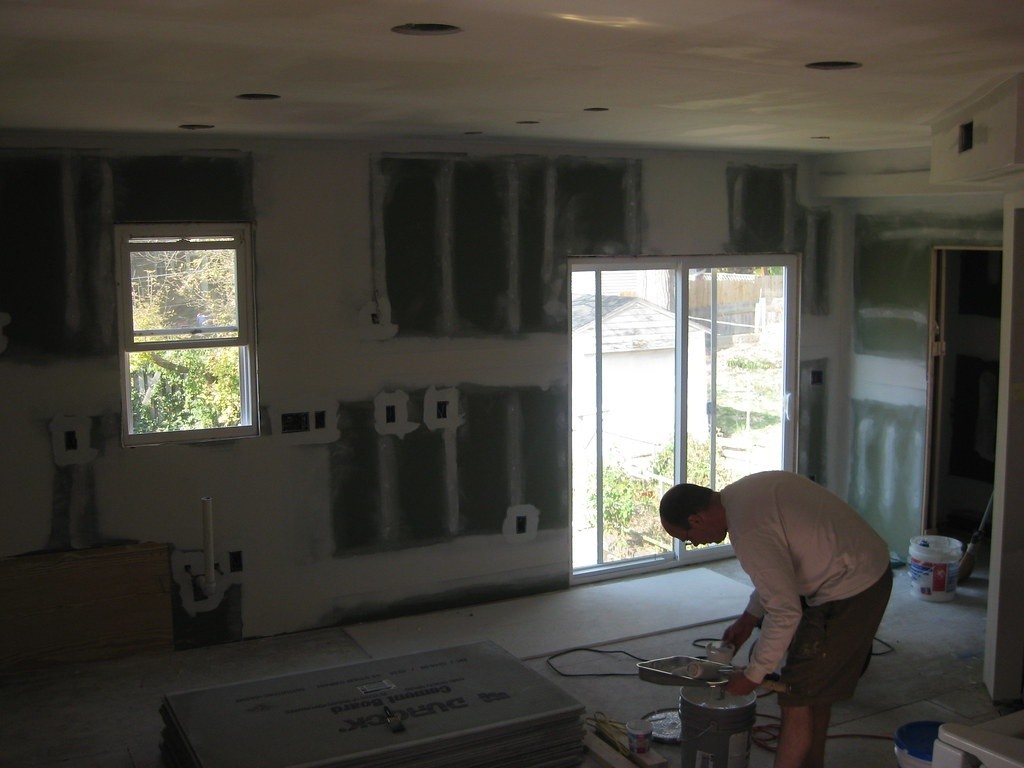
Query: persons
xmin=660 ymin=469 xmax=894 ymax=768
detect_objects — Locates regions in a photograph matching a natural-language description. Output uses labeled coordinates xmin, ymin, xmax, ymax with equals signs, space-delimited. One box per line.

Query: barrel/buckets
xmin=908 ymin=535 xmax=964 ymax=601
xmin=894 ymin=720 xmax=945 ymax=768
xmin=676 ymin=686 xmax=757 ymax=768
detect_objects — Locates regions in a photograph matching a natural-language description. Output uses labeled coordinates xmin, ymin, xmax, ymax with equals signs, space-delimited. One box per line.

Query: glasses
xmin=686 ymin=530 xmax=692 ymax=545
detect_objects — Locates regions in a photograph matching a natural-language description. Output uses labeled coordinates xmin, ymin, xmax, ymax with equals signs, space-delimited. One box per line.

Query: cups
xmin=626 ymin=720 xmax=654 ymax=753
